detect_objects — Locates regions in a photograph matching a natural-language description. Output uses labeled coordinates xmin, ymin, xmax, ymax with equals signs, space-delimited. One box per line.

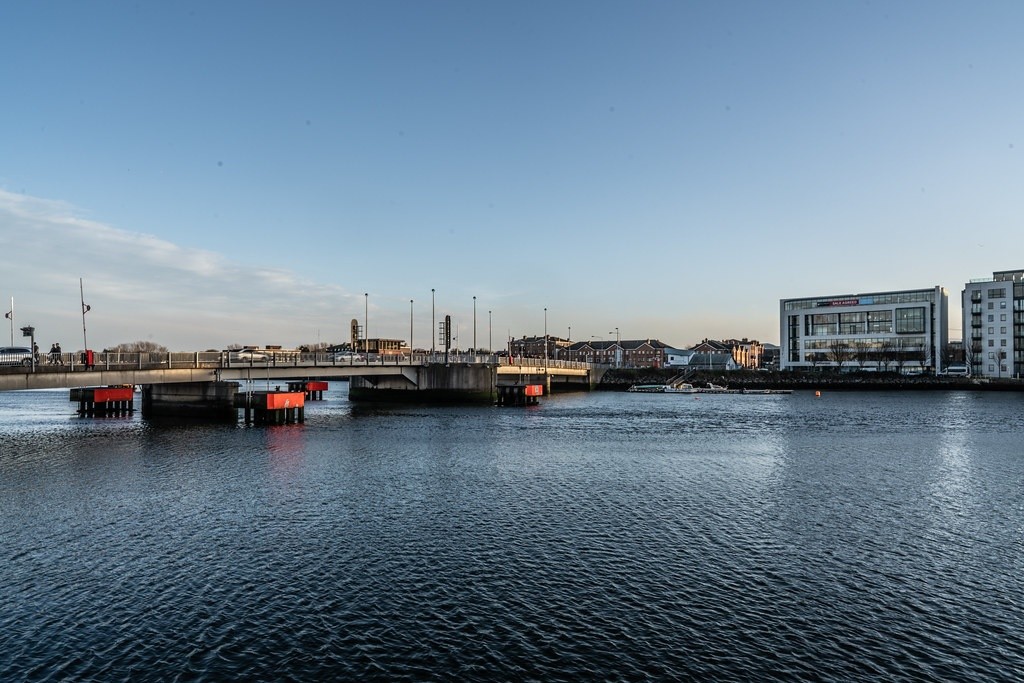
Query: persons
xmin=30 ymin=342 xmax=40 ymax=366
xmin=48 ymin=343 xmax=64 ymax=366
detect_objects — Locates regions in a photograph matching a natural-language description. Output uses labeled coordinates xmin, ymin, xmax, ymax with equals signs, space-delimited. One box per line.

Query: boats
xmin=627 ymin=382 xmax=795 ymax=394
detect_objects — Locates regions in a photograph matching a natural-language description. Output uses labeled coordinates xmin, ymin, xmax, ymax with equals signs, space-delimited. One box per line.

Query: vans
xmin=937 ymin=366 xmax=970 ymax=377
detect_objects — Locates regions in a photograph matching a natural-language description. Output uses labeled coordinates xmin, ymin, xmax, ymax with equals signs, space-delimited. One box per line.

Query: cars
xmin=0 ymin=346 xmax=36 ymax=367
xmin=328 ymin=351 xmax=362 ymax=362
xmin=907 ymin=371 xmax=921 ymax=375
xmin=369 ymin=354 xmax=381 ymax=362
xmin=231 ymin=348 xmax=270 ymax=363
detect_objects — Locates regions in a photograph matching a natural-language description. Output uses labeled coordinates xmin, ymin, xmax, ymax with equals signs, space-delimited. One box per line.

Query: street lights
xmin=608 ymin=327 xmax=619 ymax=346
xmin=473 ymin=296 xmax=476 ymax=361
xmin=591 ymin=335 xmax=603 ymax=350
xmin=432 ymin=288 xmax=435 ymax=363
xmin=569 ymin=326 xmax=571 ymax=361
xmin=544 ymin=307 xmax=548 ymax=369
xmin=489 ymin=310 xmax=491 ymax=355
xmin=410 ymin=300 xmax=413 ymax=365
xmin=365 ymin=293 xmax=369 ymax=365
